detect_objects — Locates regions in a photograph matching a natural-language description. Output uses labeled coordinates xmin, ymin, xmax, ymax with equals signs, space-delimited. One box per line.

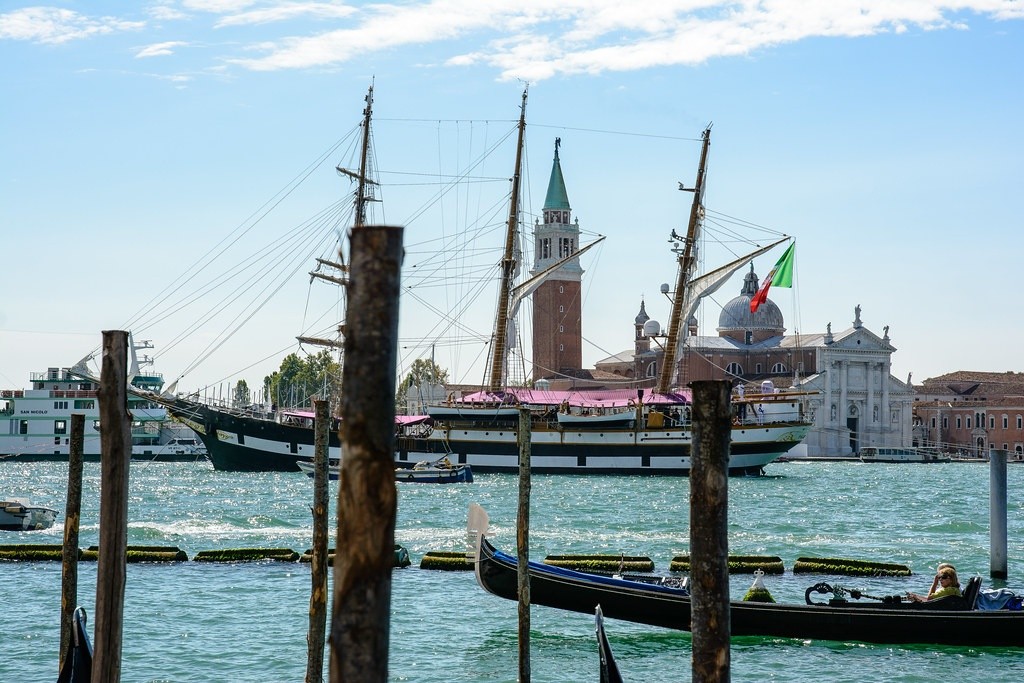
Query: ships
xmin=0 ymin=330 xmax=210 ymax=463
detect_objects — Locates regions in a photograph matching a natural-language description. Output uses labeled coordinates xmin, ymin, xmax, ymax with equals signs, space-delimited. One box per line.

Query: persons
xmin=560 ymin=399 xmax=694 ymax=428
xmin=909 ymin=562 xmax=963 ymax=603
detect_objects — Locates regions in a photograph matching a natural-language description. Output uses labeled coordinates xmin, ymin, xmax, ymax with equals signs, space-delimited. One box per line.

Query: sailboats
xmin=67 ymin=79 xmax=824 ymax=474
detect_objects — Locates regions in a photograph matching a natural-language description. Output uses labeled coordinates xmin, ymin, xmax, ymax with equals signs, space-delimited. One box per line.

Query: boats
xmin=0 ymin=501 xmax=58 ymax=532
xmin=297 ymin=414 xmax=474 ymax=483
xmin=860 ymin=446 xmax=952 ymax=464
xmin=479 ymin=535 xmax=1024 ymax=649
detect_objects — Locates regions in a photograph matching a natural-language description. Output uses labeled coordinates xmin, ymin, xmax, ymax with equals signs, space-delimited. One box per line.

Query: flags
xmin=750 ymin=242 xmax=796 ymax=311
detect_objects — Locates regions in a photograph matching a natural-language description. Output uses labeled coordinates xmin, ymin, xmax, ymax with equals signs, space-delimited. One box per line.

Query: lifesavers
xmin=732 ymin=417 xmax=741 ymax=427
